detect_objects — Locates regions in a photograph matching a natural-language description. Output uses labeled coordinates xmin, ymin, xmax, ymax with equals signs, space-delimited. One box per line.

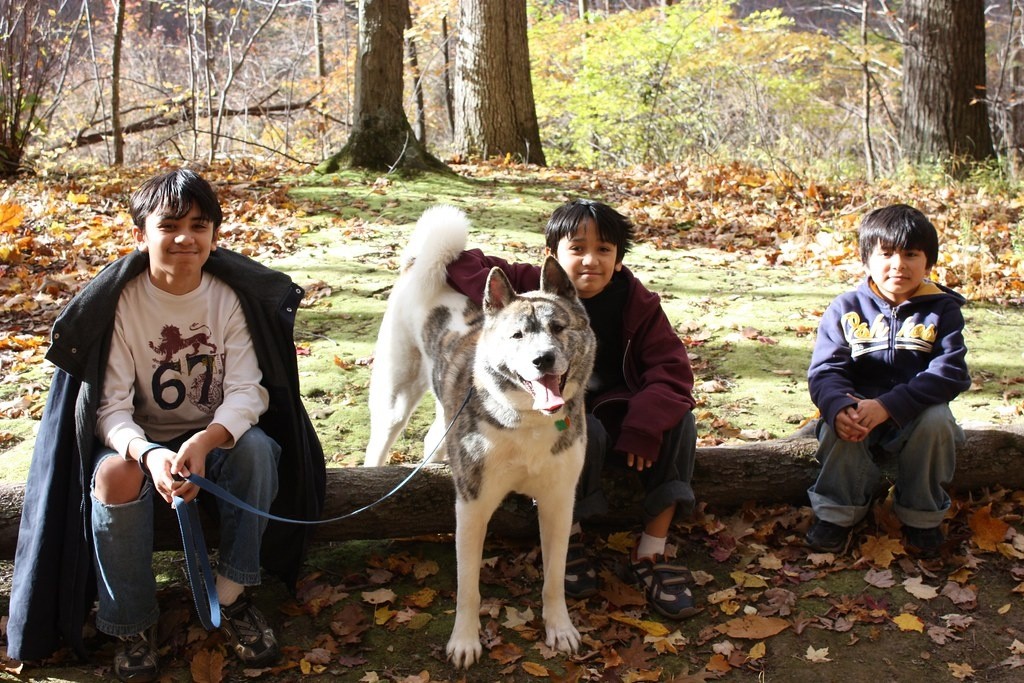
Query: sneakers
xmin=114 ymin=623 xmax=157 ymax=683
xmin=806 ymin=518 xmax=855 ymax=556
xmin=218 ymin=593 xmax=279 ymax=668
xmin=905 ymin=525 xmax=943 ymax=559
xmin=564 ymin=531 xmax=602 ymax=596
xmin=628 ymin=548 xmax=702 ymax=619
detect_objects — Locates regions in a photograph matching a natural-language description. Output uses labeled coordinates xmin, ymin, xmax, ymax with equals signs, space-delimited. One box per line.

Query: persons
xmin=86 ymin=171 xmax=281 ymax=683
xmin=445 ymin=200 xmax=697 ymax=619
xmin=807 ymin=204 xmax=971 ymax=558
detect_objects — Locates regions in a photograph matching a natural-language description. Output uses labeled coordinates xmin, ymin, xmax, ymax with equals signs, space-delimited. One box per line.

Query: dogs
xmin=363 ymin=206 xmax=597 ymax=672
